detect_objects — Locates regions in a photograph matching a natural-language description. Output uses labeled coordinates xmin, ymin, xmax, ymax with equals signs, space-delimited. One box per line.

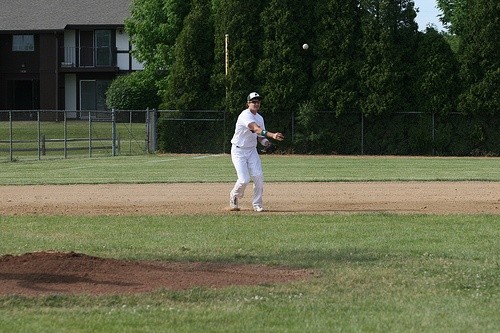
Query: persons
xmin=229 ymin=92 xmax=285 ymax=213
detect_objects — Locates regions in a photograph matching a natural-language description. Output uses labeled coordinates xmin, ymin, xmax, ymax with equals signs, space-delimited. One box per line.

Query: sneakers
xmin=251 ymin=203 xmax=266 ymax=212
xmin=229 ymin=196 xmax=241 ymax=211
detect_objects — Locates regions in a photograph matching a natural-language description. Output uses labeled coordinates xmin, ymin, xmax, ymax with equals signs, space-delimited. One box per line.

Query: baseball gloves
xmin=261 ymin=144 xmax=277 ymax=153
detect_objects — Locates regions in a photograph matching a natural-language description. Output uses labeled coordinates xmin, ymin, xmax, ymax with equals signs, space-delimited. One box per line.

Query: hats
xmin=246 ymin=91 xmax=263 ymax=101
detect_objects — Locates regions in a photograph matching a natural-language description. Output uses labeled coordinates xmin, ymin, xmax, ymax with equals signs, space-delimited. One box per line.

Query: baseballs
xmin=303 ymin=44 xmax=309 ymax=49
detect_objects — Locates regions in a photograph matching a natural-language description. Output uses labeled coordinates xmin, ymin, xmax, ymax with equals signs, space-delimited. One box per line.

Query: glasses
xmin=247 ymin=101 xmax=261 ymax=104
